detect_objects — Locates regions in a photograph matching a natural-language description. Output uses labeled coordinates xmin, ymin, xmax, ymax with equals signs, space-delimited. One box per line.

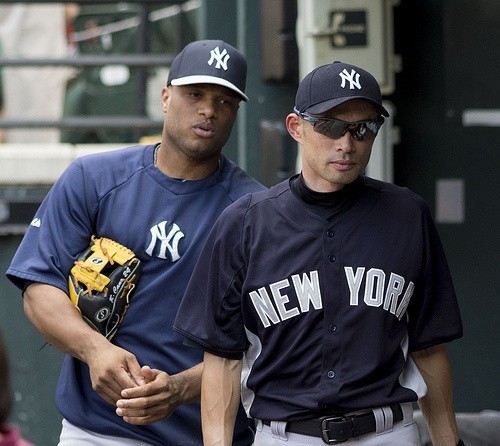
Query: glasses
xmin=294 ymin=106 xmax=384 ymax=142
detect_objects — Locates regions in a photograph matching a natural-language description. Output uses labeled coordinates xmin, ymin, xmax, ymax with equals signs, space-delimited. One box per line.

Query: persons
xmin=173 ymin=62 xmax=466 ymax=446
xmin=6 ymin=40 xmax=271 ymax=446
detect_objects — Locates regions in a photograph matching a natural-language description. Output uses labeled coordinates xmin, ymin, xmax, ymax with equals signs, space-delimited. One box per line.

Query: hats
xmin=293 ymin=61 xmax=390 ymax=118
xmin=167 ymin=40 xmax=250 ymax=103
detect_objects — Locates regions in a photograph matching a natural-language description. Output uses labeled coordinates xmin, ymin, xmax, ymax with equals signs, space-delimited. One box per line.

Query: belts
xmin=263 ymin=404 xmax=403 ymax=444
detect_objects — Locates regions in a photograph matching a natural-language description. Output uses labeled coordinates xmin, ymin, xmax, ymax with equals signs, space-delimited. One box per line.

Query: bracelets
xmin=456 ymin=439 xmax=465 ymax=446
